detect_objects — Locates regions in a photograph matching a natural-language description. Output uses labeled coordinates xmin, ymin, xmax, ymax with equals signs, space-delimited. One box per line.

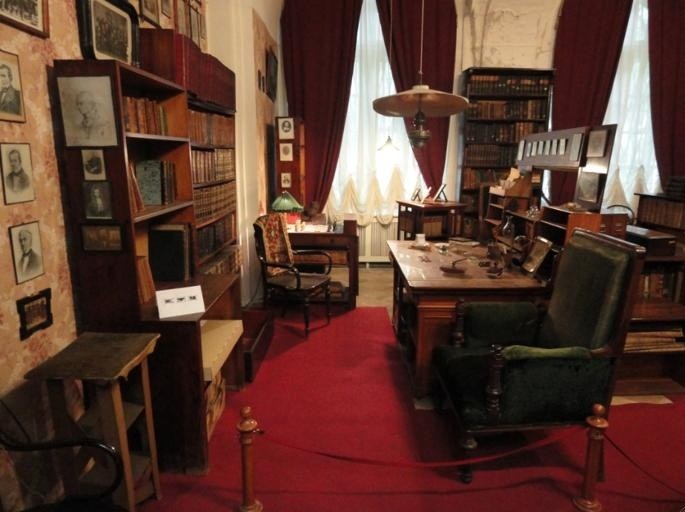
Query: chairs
xmin=0 ymin=389 xmax=126 ymax=511
xmin=416 ymin=225 xmax=644 ymax=494
xmin=251 ymin=212 xmax=334 ymax=337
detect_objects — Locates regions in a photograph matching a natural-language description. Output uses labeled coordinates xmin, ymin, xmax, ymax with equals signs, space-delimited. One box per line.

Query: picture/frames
xmin=0 ymin=1 xmax=55 ymax=42
xmin=75 ymin=1 xmax=142 ymax=65
xmin=14 ymin=288 xmax=55 ymax=344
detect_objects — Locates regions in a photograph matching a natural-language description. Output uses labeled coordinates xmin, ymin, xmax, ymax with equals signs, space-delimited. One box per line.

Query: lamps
xmin=368 ymin=0 xmax=475 ymax=150
xmin=269 ymin=189 xmax=303 ymax=230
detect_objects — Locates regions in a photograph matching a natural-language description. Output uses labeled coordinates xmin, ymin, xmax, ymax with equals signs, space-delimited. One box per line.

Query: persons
xmin=5 ymin=150 xmax=30 ymax=193
xmin=75 ymin=90 xmax=111 ymax=138
xmin=18 ymin=230 xmax=43 ymax=280
xmin=87 ymin=185 xmax=104 ymax=217
xmin=0 ymin=64 xmax=20 ymax=116
xmin=84 ymin=152 xmax=102 ymax=175
xmin=0 ymin=0 xmax=38 ymax=25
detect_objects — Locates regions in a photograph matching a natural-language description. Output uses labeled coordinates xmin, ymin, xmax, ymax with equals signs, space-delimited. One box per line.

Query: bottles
xmin=295 ymin=216 xmax=301 ymax=232
xmin=526 ymin=205 xmax=540 ymax=218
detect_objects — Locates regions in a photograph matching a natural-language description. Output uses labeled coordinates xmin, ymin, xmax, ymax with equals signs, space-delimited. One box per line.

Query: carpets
xmin=135 ymin=300 xmax=685 ymax=512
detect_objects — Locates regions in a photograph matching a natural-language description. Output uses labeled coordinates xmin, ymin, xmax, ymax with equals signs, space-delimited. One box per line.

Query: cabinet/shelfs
xmin=20 ymin=328 xmax=167 ymax=512
xmin=478 ymin=188 xmax=582 ymax=245
xmin=47 ymin=49 xmax=248 ymax=477
xmin=273 ymin=114 xmax=307 ymax=210
xmin=137 ymin=23 xmax=246 ymax=277
xmin=614 ymin=233 xmax=684 ymax=403
xmin=455 ymin=62 xmax=560 ymax=240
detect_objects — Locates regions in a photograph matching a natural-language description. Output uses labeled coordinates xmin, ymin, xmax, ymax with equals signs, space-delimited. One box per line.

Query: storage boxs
xmin=235 ymin=300 xmax=276 ymax=387
xmin=203 ymin=369 xmax=228 ymax=448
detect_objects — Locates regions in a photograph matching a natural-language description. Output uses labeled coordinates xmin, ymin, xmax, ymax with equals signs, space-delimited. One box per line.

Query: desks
xmin=380 ymin=237 xmax=552 ymax=411
xmin=389 ymin=195 xmax=469 ymax=244
xmin=283 ymin=218 xmax=363 ymax=311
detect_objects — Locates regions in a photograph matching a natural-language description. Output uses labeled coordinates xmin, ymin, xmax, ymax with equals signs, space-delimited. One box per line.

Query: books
xmin=461 ymin=75 xmax=550 ymax=239
xmin=122 ymin=96 xmax=243 ymax=283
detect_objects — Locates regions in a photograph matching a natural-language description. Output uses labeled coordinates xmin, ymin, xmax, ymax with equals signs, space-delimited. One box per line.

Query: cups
xmin=415 ymin=233 xmax=425 ymax=246
